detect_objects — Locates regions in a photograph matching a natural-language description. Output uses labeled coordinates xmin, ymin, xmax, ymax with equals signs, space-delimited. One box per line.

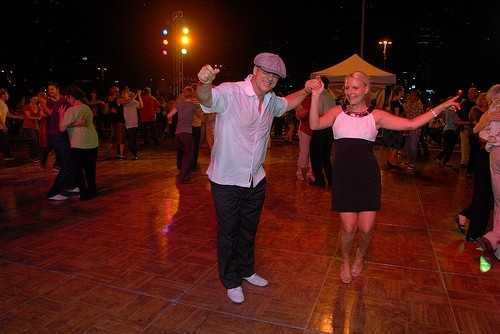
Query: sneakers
xmin=242 ymin=272 xmax=268 ymax=287
xmin=227 ymin=285 xmax=244 ymax=304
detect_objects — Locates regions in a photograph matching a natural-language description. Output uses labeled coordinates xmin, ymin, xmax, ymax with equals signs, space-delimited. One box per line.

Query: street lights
xmin=161 ymin=9 xmax=189 ymax=101
xmin=378 ymin=40 xmax=393 ymax=71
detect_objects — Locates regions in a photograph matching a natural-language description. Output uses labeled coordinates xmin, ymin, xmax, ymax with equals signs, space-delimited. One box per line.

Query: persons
xmin=166 ymin=86 xmax=204 ymax=182
xmin=197 ymin=53 xmax=321 ymax=304
xmin=14 ymin=83 xmax=110 ymax=169
xmin=109 ymin=83 xmax=217 ymax=170
xmin=0 ymin=88 xmax=25 ymax=161
xmin=57 ymin=85 xmax=99 ymax=201
xmin=380 ymin=87 xmax=458 ymax=169
xmin=455 ymin=84 xmax=500 ymax=267
xmin=268 ymin=110 xmax=299 ymax=150
xmin=310 ymin=72 xmax=461 ymax=284
xmin=45 ymin=87 xmax=88 ymax=201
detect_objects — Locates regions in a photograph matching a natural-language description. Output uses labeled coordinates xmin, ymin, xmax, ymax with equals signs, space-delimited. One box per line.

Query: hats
xmin=144 ymin=87 xmax=151 ymax=91
xmin=254 ymin=52 xmax=286 ymax=78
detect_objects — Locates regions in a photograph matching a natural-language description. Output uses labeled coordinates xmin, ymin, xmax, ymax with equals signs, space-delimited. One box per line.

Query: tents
xmin=311 ymin=54 xmax=396 ymax=117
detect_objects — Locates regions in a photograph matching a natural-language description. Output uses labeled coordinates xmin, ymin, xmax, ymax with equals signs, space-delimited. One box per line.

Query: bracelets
xmin=430 ymin=109 xmax=438 ymax=118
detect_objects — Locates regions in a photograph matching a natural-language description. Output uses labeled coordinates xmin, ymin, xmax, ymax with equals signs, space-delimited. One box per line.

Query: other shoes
xmin=466 ymin=237 xmax=478 ymax=243
xmin=340 ymin=261 xmax=352 ymax=284
xmin=38 ymin=165 xmax=44 ymax=170
xmin=452 ymin=214 xmax=467 ymax=235
xmin=104 ymin=151 xmax=110 ymax=160
xmin=66 ymin=186 xmax=80 ymax=193
xmin=306 ymin=173 xmax=315 ymax=181
xmin=53 ymin=166 xmax=60 ymax=172
xmin=479 ymin=236 xmax=497 ymax=263
xmin=352 ymin=249 xmax=367 ymax=277
xmin=30 ymin=158 xmax=40 ymax=162
xmin=79 ymin=194 xmax=96 ymax=200
xmin=433 ymin=158 xmax=443 ymax=167
xmin=119 ymin=156 xmax=126 ymax=160
xmin=308 ymin=180 xmax=326 ymax=186
xmin=131 ymin=156 xmax=137 ymax=160
xmin=296 ymin=170 xmax=304 ymax=180
xmin=47 ymin=193 xmax=69 ymax=201
xmin=446 ymin=162 xmax=452 ymax=167
